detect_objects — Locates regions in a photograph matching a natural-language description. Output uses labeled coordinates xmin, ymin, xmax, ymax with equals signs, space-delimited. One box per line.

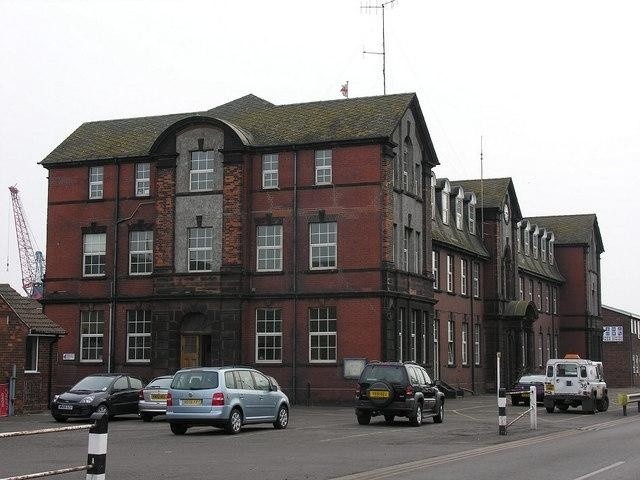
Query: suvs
xmin=544 ymin=354 xmax=608 ymax=414
xmin=352 ymin=361 xmax=445 ymax=426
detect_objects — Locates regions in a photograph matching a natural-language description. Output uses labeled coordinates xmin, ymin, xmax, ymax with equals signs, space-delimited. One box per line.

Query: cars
xmin=51 ymin=366 xmax=290 ymax=434
xmin=512 ymin=374 xmax=546 ymax=405
